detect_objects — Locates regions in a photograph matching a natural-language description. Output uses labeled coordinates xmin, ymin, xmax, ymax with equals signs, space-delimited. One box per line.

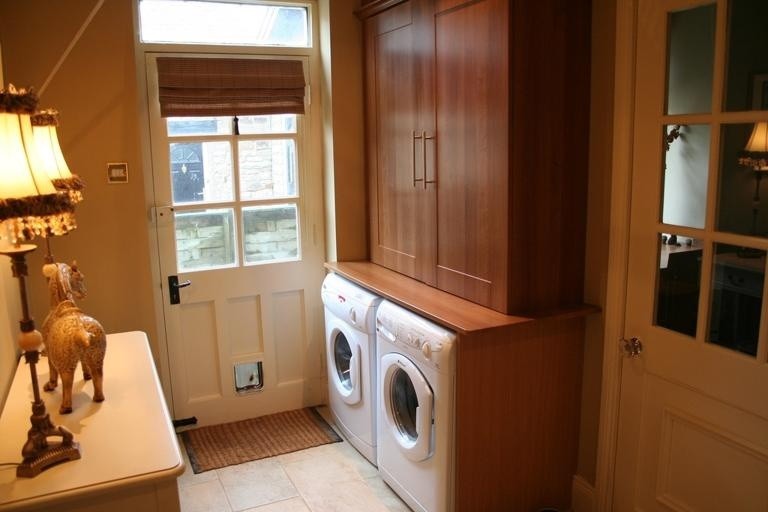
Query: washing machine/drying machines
xmin=377 ymin=297 xmax=456 ymax=512
xmin=320 ymin=271 xmax=381 ymax=469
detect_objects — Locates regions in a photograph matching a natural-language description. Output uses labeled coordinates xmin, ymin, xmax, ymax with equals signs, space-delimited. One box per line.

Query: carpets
xmin=178 ymin=404 xmax=344 ymax=473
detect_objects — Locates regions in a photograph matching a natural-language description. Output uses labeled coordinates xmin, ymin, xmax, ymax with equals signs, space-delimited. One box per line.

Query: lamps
xmin=25 ymin=99 xmax=83 ymax=262
xmin=735 ymin=122 xmax=768 ymax=254
xmin=0 ymin=83 xmax=80 ymax=477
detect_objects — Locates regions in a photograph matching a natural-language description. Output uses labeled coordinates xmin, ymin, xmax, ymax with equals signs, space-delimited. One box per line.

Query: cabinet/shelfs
xmin=701 ymin=248 xmax=768 ymax=342
xmin=2 ymin=329 xmax=185 ymax=510
xmin=362 ymin=0 xmax=593 ymax=312
